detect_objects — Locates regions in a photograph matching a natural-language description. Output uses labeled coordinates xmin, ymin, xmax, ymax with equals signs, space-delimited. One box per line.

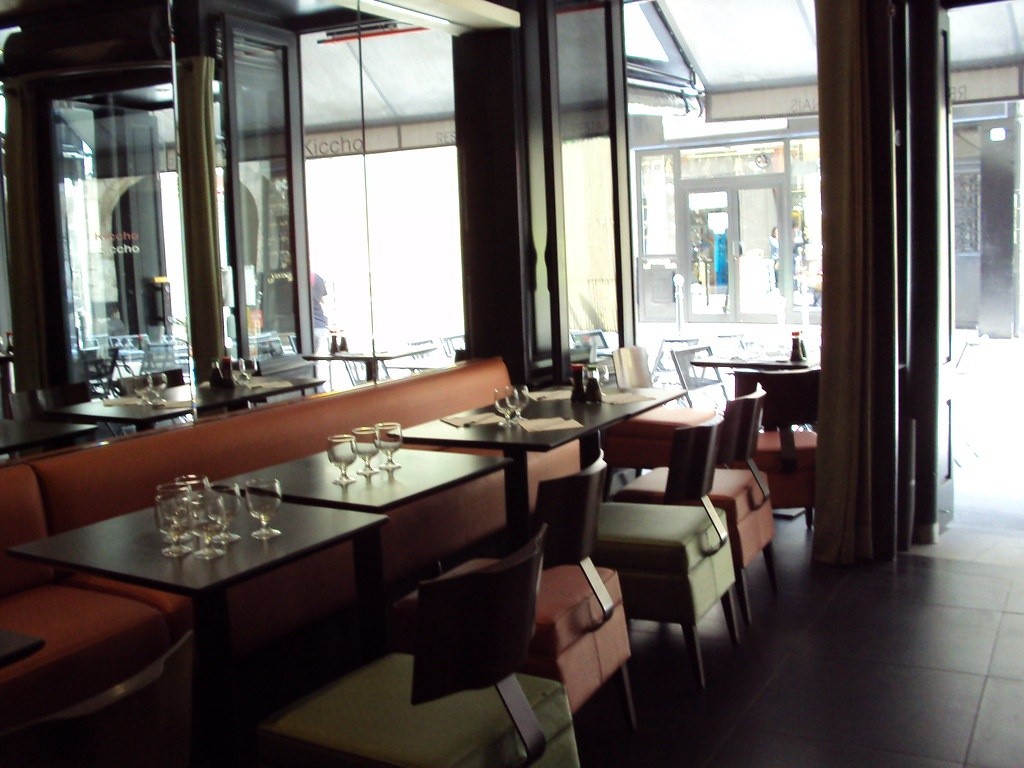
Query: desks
xmin=1 ymin=423 xmax=98 ymax=455
xmin=2 ymin=486 xmax=388 ymax=768
xmin=41 ymin=373 xmax=327 ymax=433
xmin=386 ymin=384 xmax=689 ymax=524
xmin=302 ymin=344 xmax=436 ymax=382
xmin=205 ymin=441 xmax=514 ymax=631
xmin=690 ymin=358 xmax=818 ymax=396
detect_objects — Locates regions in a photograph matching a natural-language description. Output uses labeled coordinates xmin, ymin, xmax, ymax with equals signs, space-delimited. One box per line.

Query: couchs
xmin=32 ymin=361 xmax=580 ymax=667
xmin=0 ymin=464 xmax=188 ymax=764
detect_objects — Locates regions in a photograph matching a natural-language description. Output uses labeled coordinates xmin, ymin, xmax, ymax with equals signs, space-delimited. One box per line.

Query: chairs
xmin=729 ymin=370 xmax=818 ymax=528
xmin=407 ymin=450 xmax=641 ymax=747
xmin=598 ymin=425 xmax=740 ymax=688
xmin=616 ymin=388 xmax=777 ymax=628
xmin=573 ymin=329 xmax=752 ymax=409
xmin=0 ymin=333 xmax=300 ymax=439
xmin=256 ymin=521 xmax=582 ymax=768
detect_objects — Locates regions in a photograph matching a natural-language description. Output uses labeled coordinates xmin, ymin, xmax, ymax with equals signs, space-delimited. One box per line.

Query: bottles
xmin=144 ymin=334 xmax=150 ymax=349
xmin=139 ymin=335 xmax=143 ymax=349
xmin=800 ymin=331 xmax=807 ymax=360
xmin=792 ymin=332 xmax=803 ymax=361
xmin=331 ymin=329 xmax=338 ymax=354
xmin=339 ymin=329 xmax=348 ymax=353
xmin=220 ymin=357 xmax=234 ymax=388
xmin=6 ymin=331 xmax=14 ymax=355
xmin=585 ymin=366 xmax=602 ymax=404
xmin=211 ymin=357 xmax=222 ymax=386
xmin=570 ymin=364 xmax=585 ymax=403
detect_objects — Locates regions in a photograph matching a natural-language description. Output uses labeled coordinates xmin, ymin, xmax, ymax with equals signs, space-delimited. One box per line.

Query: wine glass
xmin=506 ymin=385 xmax=530 ymax=424
xmin=0 ymin=336 xmax=6 ymax=357
xmin=153 ymin=475 xmax=283 ymax=560
xmin=351 ymin=427 xmax=380 ymax=475
xmin=240 ymin=358 xmax=258 ymax=387
xmin=494 ymin=387 xmax=519 ymax=427
xmin=148 ymin=373 xmax=168 ymax=405
xmin=231 ymin=361 xmax=242 ymax=387
xmin=326 ymin=434 xmax=359 ymax=484
xmin=373 ymin=422 xmax=403 ymax=470
xmin=133 ymin=373 xmax=152 ymax=406
xmin=596 ymin=365 xmax=609 ymax=397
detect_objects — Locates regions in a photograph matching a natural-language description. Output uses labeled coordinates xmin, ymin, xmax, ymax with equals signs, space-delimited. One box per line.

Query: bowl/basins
xmin=288 ymin=377 xmax=313 ymax=384
